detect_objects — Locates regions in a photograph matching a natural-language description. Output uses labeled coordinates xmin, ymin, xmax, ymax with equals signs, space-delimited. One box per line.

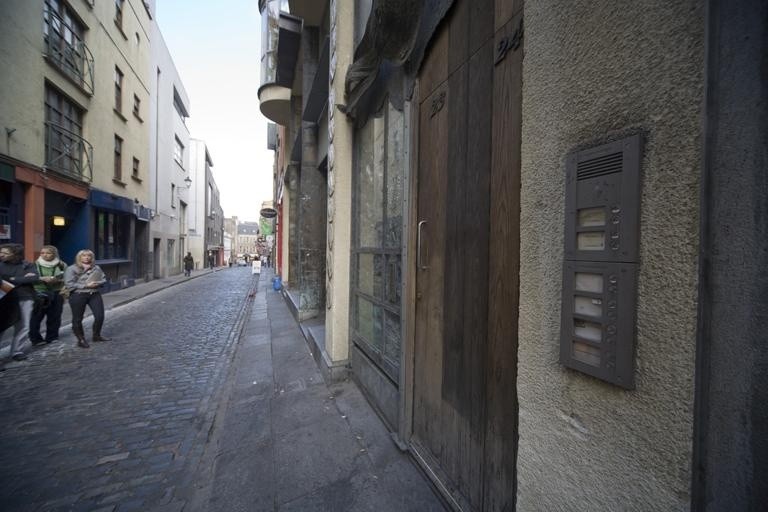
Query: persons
xmin=29 ymin=245 xmax=68 ymax=346
xmin=64 ymin=249 xmax=112 ymax=348
xmin=183 ymin=252 xmax=194 ymax=277
xmin=0 ymin=243 xmax=39 ymax=371
xmin=228 ymin=254 xmax=233 ymax=267
xmin=208 ymin=253 xmax=214 ymax=270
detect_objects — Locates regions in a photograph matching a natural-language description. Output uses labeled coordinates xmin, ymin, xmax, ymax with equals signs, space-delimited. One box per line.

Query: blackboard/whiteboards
xmin=252 ymin=261 xmax=261 ymax=273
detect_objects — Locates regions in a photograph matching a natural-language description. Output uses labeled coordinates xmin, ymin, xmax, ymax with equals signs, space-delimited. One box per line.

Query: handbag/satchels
xmin=35 ymin=292 xmax=50 ymax=309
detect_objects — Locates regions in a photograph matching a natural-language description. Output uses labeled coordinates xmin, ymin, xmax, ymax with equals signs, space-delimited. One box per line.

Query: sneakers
xmin=32 ymin=337 xmax=60 ymax=346
xmin=93 ymin=335 xmax=112 ymax=341
xmin=10 ymin=352 xmax=27 ymax=361
xmin=78 ymin=338 xmax=90 ymax=348
